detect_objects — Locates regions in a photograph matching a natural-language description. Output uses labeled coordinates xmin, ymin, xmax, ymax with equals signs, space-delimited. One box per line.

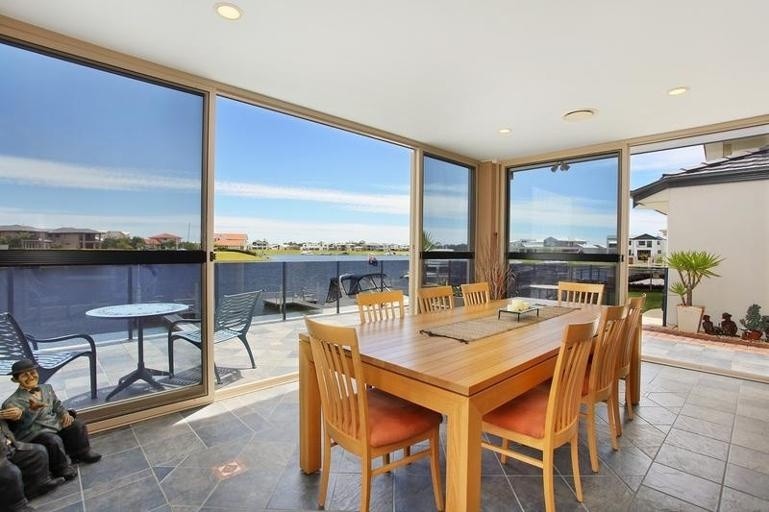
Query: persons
xmin=1 ymin=404 xmax=68 ymax=512
xmin=719 ymin=312 xmax=738 ymax=336
xmin=3 ymin=357 xmax=103 ymax=483
xmin=701 ymin=314 xmax=717 ymax=336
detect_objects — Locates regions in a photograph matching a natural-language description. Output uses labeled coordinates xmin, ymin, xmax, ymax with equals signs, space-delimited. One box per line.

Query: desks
xmin=82 ymin=300 xmax=195 ymax=403
xmin=294 ymin=292 xmax=643 ymax=512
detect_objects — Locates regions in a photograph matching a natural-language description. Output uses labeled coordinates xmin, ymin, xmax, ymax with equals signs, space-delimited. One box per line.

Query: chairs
xmin=480 ymin=313 xmax=600 ymax=512
xmin=622 ymin=291 xmax=641 ymax=425
xmin=415 ymin=283 xmax=457 ymax=316
xmin=459 ymin=280 xmax=490 ymax=309
xmin=158 ymin=286 xmax=267 ymax=386
xmin=303 ymin=309 xmax=443 ymax=511
xmin=541 ymin=304 xmax=634 ymax=472
xmin=0 ymin=307 xmax=100 ymax=401
xmin=554 ymin=279 xmax=605 ymax=305
xmin=355 ymin=289 xmax=412 ymax=467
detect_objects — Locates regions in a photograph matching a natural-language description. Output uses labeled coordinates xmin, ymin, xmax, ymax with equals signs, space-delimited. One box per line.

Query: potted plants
xmin=738 ymin=304 xmax=764 ymax=341
xmin=666 ymin=247 xmax=728 ymax=334
